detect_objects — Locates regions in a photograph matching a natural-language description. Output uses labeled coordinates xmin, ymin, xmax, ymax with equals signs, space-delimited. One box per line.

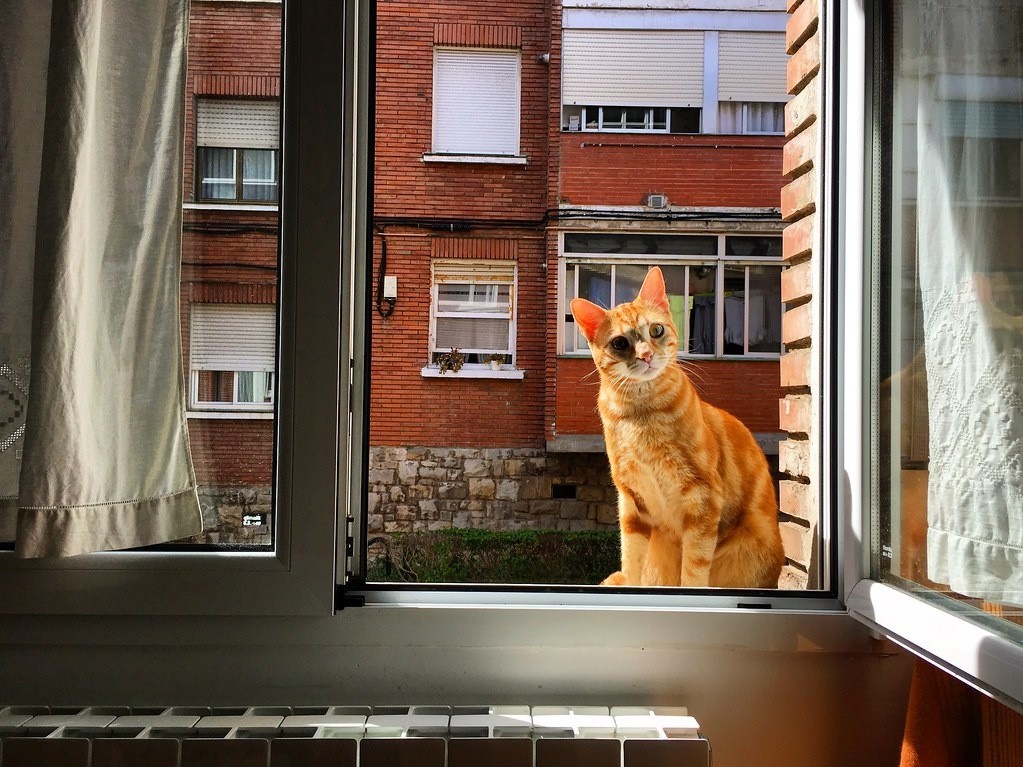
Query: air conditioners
xmin=650 ymin=195 xmax=664 ymax=208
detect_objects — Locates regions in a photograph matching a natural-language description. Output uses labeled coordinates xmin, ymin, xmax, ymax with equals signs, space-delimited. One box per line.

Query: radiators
xmin=0 ymin=704 xmax=713 ymax=767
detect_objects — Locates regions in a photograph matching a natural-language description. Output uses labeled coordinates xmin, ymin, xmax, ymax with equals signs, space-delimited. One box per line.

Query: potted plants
xmin=435 ymin=346 xmax=464 ymax=374
xmin=484 ymin=353 xmax=504 ymax=370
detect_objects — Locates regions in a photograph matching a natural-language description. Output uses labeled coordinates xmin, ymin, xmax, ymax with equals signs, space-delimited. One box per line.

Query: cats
xmin=570 ymin=266 xmax=786 ymax=588
